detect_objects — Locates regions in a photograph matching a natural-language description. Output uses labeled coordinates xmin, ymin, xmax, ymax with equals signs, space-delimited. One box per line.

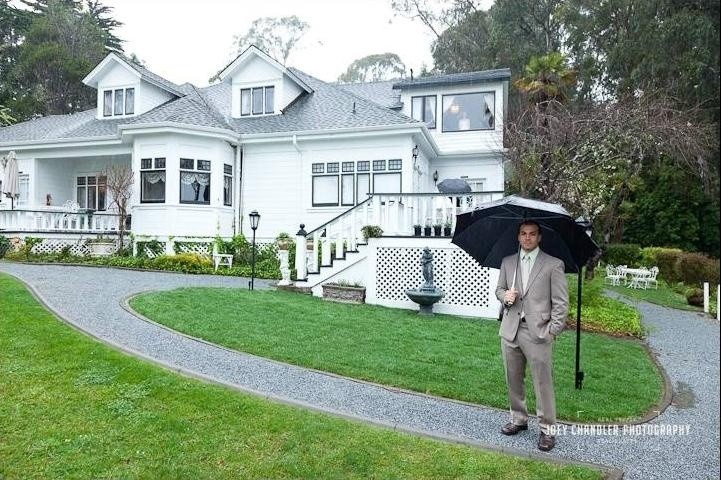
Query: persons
xmin=459 ymin=113 xmax=470 ymax=130
xmin=420 ymin=248 xmax=433 ymax=288
xmin=495 ymin=220 xmax=569 ymax=450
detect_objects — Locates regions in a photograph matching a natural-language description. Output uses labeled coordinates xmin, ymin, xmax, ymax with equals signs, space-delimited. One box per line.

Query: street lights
xmin=249 ymin=209 xmax=261 ymax=290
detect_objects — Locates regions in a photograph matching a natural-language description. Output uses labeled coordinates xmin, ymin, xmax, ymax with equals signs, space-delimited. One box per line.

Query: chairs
xmin=604 ymin=263 xmax=659 ymax=290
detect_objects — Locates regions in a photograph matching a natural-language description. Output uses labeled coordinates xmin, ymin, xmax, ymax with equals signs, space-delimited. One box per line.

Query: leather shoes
xmin=537 ymin=431 xmax=555 ymax=451
xmin=502 ymin=422 xmax=528 ymax=435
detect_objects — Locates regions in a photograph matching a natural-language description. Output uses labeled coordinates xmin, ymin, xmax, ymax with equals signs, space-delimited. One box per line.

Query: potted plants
xmin=424 ymin=226 xmax=431 ymax=236
xmin=322 ymin=281 xmax=365 ymax=303
xmin=413 ymin=224 xmax=422 ymax=235
xmin=443 ymin=222 xmax=451 ymax=236
xmin=433 ymin=224 xmax=441 ymax=236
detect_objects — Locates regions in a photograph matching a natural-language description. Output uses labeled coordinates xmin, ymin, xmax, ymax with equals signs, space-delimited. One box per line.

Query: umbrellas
xmin=451 ymin=194 xmax=601 ymax=305
xmin=0 ymin=151 xmax=20 ymax=211
xmin=438 ymin=179 xmax=471 ymax=195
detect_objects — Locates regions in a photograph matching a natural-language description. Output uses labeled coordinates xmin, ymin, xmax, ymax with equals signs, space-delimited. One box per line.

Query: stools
xmin=213 ymin=254 xmax=233 ymax=270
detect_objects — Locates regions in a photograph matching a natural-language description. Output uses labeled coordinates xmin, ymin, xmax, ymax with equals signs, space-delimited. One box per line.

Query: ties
xmin=520 ymin=254 xmax=530 ymax=319
